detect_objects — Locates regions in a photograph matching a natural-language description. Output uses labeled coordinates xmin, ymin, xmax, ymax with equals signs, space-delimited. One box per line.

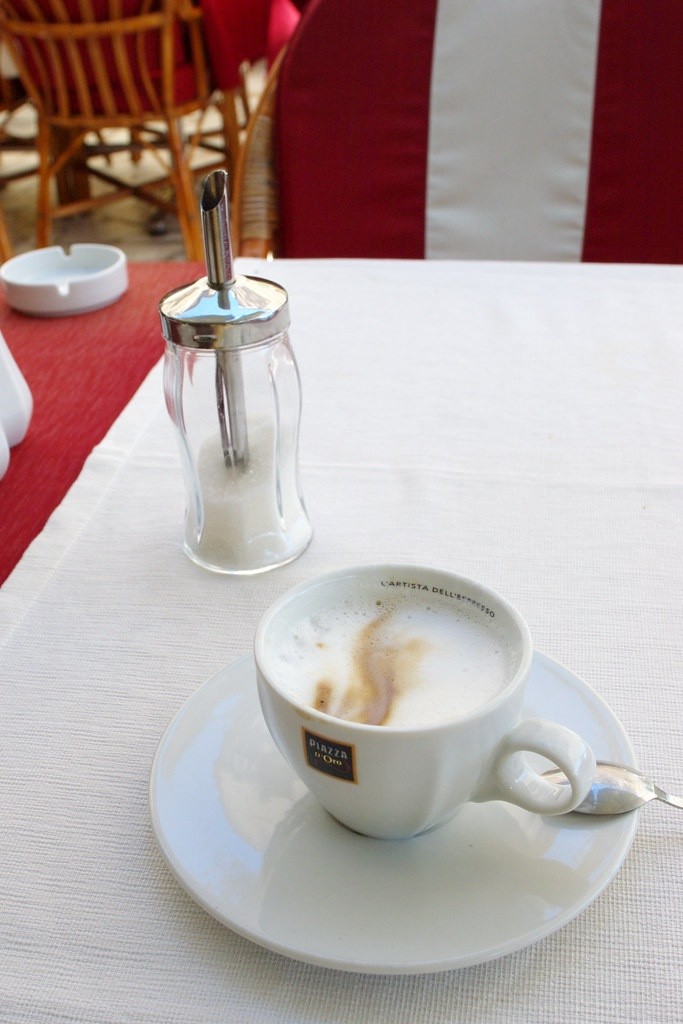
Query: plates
xmin=149 ymin=651 xmax=641 ymax=975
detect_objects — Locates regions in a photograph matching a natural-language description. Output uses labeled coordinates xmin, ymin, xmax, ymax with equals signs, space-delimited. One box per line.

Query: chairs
xmin=229 ymin=0 xmax=683 ymax=264
xmin=0 ymin=0 xmax=253 ymax=250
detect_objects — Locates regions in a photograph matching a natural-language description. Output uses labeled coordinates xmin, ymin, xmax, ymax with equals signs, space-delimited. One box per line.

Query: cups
xmin=254 ymin=564 xmax=596 ymax=839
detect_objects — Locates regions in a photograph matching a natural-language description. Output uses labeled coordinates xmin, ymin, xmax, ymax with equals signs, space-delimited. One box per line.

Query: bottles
xmin=159 ymin=275 xmax=314 ymax=575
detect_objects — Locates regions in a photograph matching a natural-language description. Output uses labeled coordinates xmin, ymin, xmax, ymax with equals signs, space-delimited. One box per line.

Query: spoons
xmin=538 ymin=762 xmax=683 ymax=817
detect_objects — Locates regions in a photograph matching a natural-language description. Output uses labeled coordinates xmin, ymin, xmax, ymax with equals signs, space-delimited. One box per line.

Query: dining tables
xmin=0 ymin=262 xmax=683 ymax=1024
